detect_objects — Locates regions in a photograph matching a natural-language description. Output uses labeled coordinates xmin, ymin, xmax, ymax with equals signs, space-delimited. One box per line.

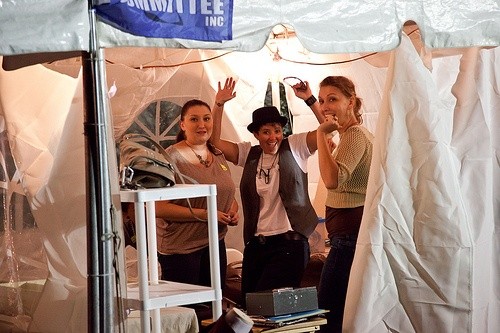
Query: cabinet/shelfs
xmin=0 ymin=183 xmax=222 ymax=333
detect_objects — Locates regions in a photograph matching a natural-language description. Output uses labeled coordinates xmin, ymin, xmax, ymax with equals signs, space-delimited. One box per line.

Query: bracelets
xmin=215 ymin=100 xmax=225 ymax=107
xmin=304 ymin=94 xmax=317 ymax=107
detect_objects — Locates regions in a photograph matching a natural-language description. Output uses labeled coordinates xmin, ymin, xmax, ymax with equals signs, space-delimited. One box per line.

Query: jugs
xmin=309 ymin=216 xmax=326 ymax=253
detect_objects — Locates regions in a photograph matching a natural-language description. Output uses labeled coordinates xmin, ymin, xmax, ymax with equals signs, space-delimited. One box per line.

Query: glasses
xmin=283 ymin=77 xmax=307 ymax=92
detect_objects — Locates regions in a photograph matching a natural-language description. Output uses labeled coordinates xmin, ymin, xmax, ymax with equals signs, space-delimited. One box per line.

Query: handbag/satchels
xmin=115 ymin=133 xmax=176 ymax=188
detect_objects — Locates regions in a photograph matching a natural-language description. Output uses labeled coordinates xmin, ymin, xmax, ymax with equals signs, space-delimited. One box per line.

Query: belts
xmin=250 ymin=231 xmax=305 ymax=246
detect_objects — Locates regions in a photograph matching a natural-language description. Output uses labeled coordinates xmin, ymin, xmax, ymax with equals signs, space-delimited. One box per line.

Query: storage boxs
xmin=245 ymin=286 xmax=318 ymax=316
xmin=308 ymin=217 xmax=326 ymax=253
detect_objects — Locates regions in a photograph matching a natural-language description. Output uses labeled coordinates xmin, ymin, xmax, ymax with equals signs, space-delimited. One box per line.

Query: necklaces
xmin=344 ymin=121 xmax=359 ymax=133
xmin=184 ymin=140 xmax=211 ymax=167
xmin=259 ymin=147 xmax=279 ymax=184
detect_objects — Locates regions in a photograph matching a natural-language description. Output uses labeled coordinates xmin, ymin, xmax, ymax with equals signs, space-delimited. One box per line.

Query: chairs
xmin=226 ymin=249 xmax=243 ymax=278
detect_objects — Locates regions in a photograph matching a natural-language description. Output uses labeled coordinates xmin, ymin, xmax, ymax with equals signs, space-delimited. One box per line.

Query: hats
xmin=247 ymin=106 xmax=287 ymax=133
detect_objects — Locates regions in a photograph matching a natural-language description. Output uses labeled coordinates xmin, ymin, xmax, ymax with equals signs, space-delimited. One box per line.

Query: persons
xmin=208 ymin=77 xmax=339 ymax=311
xmin=316 ymin=76 xmax=375 ymax=333
xmin=141 ymin=100 xmax=241 ymax=312
